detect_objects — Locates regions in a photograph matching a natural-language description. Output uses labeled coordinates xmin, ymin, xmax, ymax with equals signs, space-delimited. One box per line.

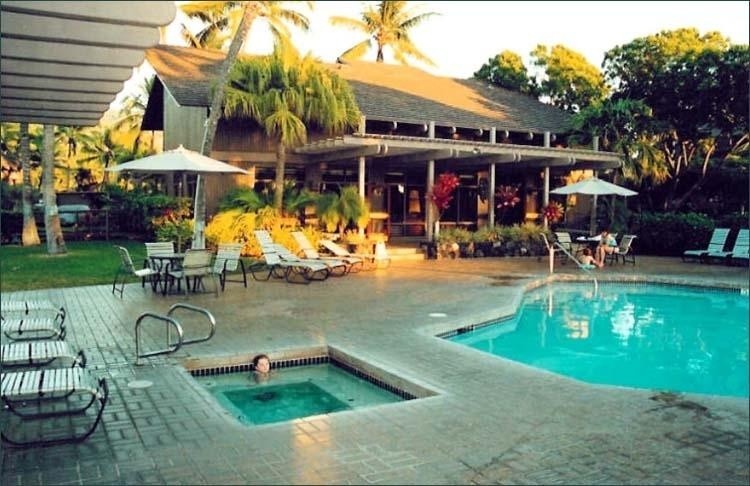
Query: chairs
xmin=197 ymin=245 xmax=246 ymax=291
xmin=112 ymin=247 xmax=163 ymax=299
xmin=682 ymin=228 xmax=731 ymax=264
xmin=164 ymin=248 xmax=218 ymax=300
xmin=539 ymin=232 xmax=637 ymax=273
xmin=142 ymin=242 xmax=190 ymax=294
xmin=249 ymin=229 xmax=395 ymax=284
xmin=1 ymin=299 xmax=109 ymax=445
xmin=707 ymin=229 xmax=750 ymax=267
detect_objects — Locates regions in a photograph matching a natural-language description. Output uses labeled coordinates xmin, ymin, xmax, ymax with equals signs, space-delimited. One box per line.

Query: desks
xmin=149 ymin=253 xmax=185 ymax=291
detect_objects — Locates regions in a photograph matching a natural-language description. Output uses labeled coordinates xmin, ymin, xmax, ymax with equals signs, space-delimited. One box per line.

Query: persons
xmin=578 ymin=249 xmax=600 ymax=270
xmin=595 ymin=228 xmax=620 ymax=267
xmin=253 ymin=355 xmax=270 ymax=373
xmin=254 ymin=373 xmax=270 ymax=384
xmin=581 ymin=287 xmax=623 ymax=314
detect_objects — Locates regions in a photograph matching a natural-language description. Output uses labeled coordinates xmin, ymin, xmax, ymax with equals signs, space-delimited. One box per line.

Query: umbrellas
xmin=108 ymin=143 xmax=252 ymax=254
xmin=550 ymin=176 xmax=639 ymax=235
